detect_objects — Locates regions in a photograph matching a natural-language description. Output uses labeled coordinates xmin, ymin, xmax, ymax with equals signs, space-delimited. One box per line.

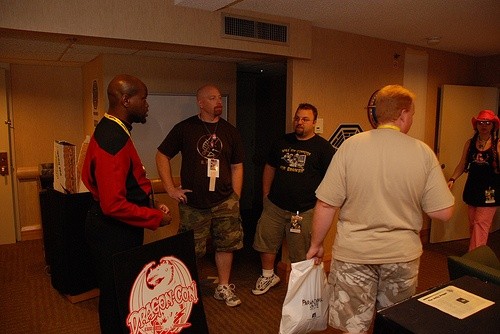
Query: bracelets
xmin=449 ymin=178 xmax=455 ymax=182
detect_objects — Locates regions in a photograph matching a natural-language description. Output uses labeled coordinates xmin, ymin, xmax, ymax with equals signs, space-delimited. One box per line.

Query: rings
xmin=179 ymin=197 xmax=183 ymax=199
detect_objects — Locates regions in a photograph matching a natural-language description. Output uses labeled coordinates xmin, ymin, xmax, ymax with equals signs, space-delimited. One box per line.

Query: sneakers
xmin=213 ymin=283 xmax=242 ymax=307
xmin=252 ymin=272 xmax=281 ymax=295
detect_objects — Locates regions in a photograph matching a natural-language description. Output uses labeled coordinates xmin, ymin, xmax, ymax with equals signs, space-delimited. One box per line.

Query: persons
xmin=81 ymin=74 xmax=172 ymax=334
xmin=307 ymin=85 xmax=455 ymax=334
xmin=447 ymin=109 xmax=500 ymax=252
xmin=252 ymin=103 xmax=336 ymax=295
xmin=156 ymin=84 xmax=246 ymax=307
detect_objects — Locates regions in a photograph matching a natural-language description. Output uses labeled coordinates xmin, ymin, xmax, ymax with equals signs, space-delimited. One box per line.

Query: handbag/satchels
xmin=278 ymin=257 xmax=330 ymax=334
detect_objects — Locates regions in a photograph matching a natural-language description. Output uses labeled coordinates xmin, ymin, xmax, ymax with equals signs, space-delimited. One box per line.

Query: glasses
xmin=477 ymin=120 xmax=493 ymax=126
xmin=293 ymin=116 xmax=312 ymax=123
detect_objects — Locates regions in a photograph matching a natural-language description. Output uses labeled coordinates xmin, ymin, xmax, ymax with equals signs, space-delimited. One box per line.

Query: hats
xmin=471 ymin=110 xmax=500 ymax=132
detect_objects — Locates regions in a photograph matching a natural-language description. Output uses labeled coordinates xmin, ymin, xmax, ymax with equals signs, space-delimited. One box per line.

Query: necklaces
xmin=201 ymin=116 xmax=218 ymax=138
